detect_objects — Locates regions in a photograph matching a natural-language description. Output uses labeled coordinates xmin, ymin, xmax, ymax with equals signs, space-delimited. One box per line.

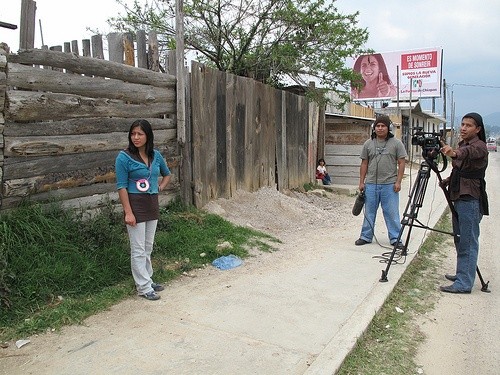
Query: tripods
xmin=380 ymin=160 xmax=491 ymax=294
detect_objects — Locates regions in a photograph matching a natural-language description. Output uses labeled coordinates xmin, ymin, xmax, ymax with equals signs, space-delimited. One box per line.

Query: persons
xmin=440 ymin=112 xmax=490 ymax=294
xmin=354 ymin=115 xmax=407 ymax=249
xmin=317 ymin=159 xmax=332 ymax=185
xmin=116 ymin=119 xmax=172 ymax=300
xmin=351 ymin=53 xmax=396 ymax=99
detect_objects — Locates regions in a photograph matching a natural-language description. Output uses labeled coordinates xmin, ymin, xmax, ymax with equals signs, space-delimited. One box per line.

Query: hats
xmin=373 ymin=116 xmax=391 ymax=130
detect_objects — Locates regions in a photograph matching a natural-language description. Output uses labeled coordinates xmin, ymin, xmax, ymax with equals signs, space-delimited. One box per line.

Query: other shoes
xmin=391 ymin=241 xmax=403 ymax=248
xmin=355 ymin=238 xmax=371 ymax=245
xmin=151 ymin=283 xmax=164 ymax=291
xmin=445 ymin=273 xmax=457 ymax=282
xmin=440 ymin=286 xmax=471 ymax=294
xmin=139 ymin=291 xmax=160 ymax=300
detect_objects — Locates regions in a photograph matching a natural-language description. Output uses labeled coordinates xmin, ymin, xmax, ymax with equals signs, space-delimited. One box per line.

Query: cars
xmin=487 ymin=137 xmax=497 ymax=152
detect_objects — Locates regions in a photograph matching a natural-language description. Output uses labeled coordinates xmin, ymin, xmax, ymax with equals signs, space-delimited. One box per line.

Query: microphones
xmin=352 ymin=191 xmax=366 ymax=216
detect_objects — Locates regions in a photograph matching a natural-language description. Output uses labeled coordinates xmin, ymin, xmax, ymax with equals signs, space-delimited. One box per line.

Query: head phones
xmin=370 ymin=115 xmax=394 ymax=130
